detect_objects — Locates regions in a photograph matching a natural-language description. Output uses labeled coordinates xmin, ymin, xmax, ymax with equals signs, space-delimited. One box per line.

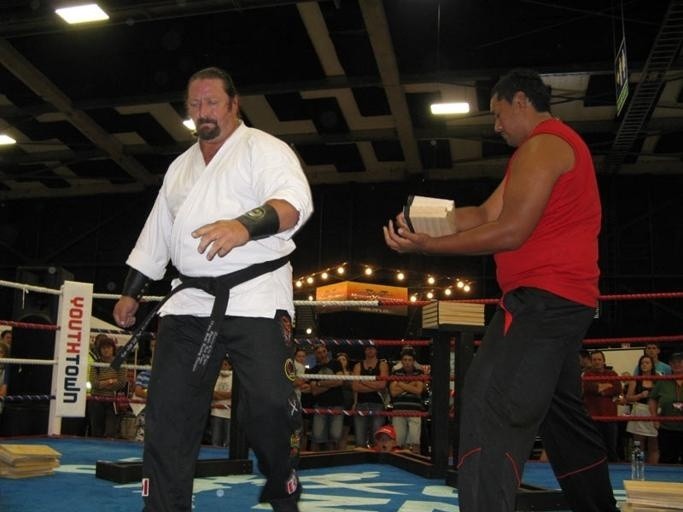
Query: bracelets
xmin=235 ymin=205 xmax=281 ymax=241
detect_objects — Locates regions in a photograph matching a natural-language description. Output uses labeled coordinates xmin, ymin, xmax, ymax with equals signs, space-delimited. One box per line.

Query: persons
xmin=210 ymin=358 xmax=232 ymax=448
xmin=380 ymin=65 xmax=623 ymax=512
xmin=108 ymin=66 xmax=317 ymax=512
xmin=295 ymin=341 xmax=456 ymax=458
xmin=542 ymin=341 xmax=683 ymax=464
xmin=0 ymin=313 xmax=81 ymax=435
xmin=86 ymin=333 xmax=158 ymax=443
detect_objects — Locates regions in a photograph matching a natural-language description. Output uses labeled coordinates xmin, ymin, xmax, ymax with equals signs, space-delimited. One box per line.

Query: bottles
xmin=631 ymin=441 xmax=644 ymax=480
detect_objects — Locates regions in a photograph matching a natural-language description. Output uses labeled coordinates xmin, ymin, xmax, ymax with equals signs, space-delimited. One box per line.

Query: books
xmin=620 ymin=478 xmax=683 ymax=512
